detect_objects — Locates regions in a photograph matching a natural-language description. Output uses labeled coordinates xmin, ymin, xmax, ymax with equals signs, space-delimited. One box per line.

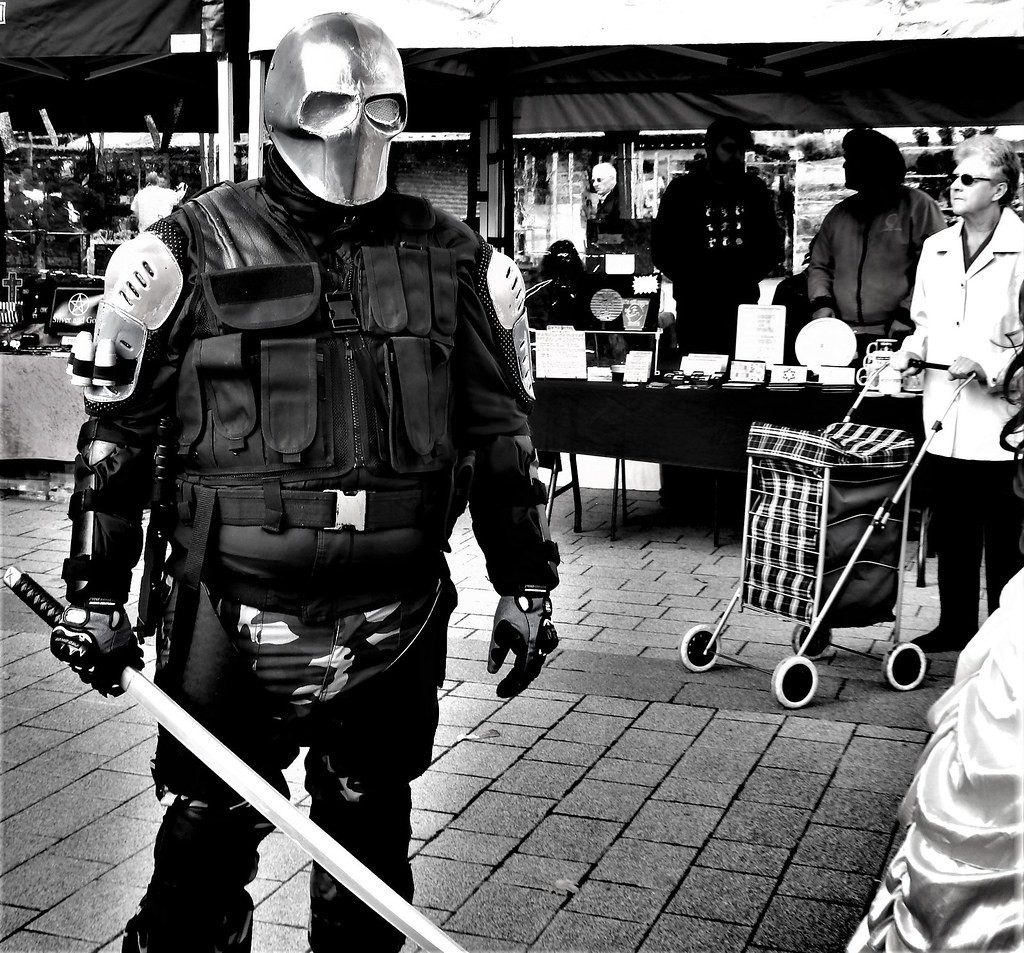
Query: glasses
xmin=945 ymin=173 xmax=999 ymax=186
xmin=591 ymin=178 xmax=608 ymax=183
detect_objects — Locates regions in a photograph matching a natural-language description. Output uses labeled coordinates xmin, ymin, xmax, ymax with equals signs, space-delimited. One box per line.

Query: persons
xmin=889 ymin=134 xmax=1024 ymax=653
xmin=130 ymin=171 xmax=189 ymax=234
xmin=592 ymin=162 xmax=620 ymax=220
xmin=50 ymin=12 xmax=560 ymax=953
xmin=807 ymin=128 xmax=948 ymax=356
xmin=650 ymin=118 xmax=785 ymax=355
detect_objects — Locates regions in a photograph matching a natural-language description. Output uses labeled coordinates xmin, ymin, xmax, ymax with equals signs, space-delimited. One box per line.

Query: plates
xmin=795 ymin=317 xmax=859 ymax=373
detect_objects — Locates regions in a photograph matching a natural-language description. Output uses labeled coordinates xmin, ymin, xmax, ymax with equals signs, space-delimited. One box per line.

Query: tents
xmin=248 ymin=0 xmax=1023 ymax=261
xmin=0 ymin=0 xmax=488 ymax=242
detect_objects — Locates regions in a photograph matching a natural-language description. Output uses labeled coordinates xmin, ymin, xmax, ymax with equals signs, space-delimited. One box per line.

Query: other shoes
xmin=908 ymin=621 xmax=981 ymax=654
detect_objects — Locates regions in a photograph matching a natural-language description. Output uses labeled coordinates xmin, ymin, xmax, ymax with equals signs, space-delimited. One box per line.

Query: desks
xmin=531 ymin=372 xmax=921 ymax=542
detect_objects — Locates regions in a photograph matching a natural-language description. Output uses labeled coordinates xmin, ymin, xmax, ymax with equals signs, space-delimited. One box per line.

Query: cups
xmin=856 ymin=338 xmax=925 ymax=394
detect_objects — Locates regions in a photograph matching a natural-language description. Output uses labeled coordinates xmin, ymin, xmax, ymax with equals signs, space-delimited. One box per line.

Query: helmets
xmin=262 ymin=11 xmax=408 ymax=208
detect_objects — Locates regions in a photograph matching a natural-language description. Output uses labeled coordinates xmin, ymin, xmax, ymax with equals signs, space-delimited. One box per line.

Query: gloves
xmin=50 ymin=598 xmax=145 ymax=698
xmin=488 ymin=596 xmax=554 ymax=701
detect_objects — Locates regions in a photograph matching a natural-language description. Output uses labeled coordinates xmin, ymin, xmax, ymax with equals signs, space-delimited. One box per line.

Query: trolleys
xmin=680 ymin=361 xmax=974 ymax=707
